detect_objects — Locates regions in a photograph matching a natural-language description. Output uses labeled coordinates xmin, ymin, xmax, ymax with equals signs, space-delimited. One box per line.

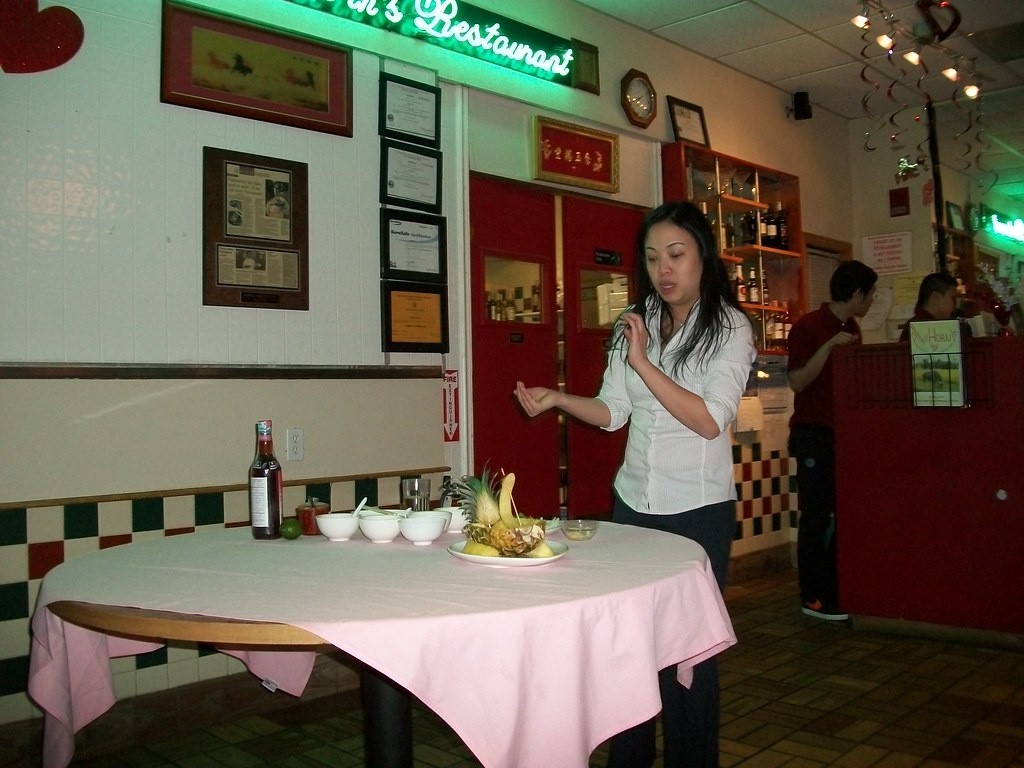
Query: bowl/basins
xmin=316 ymin=507 xmax=471 ymax=546
xmin=560 ymin=520 xmax=599 ymax=541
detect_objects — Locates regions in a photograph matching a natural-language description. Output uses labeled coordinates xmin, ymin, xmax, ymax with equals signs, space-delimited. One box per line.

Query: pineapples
xmin=462 ymin=472 xmax=554 ymax=559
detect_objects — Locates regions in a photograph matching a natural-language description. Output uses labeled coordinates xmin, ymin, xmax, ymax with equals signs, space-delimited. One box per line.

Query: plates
xmin=447 ymin=541 xmax=570 ymax=567
xmin=544 ymin=521 xmax=562 ymax=533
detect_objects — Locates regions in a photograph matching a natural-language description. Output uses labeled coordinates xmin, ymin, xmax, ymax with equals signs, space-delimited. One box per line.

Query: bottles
xmin=249 ymin=420 xmax=283 ymax=541
xmin=705 ymin=201 xmax=792 ymax=351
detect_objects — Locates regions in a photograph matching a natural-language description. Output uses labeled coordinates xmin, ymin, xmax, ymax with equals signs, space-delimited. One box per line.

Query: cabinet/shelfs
xmin=932 ymin=224 xmax=961 ymax=271
xmin=512 ymin=309 xmax=567 ymax=507
xmin=660 ymin=143 xmax=808 ymax=354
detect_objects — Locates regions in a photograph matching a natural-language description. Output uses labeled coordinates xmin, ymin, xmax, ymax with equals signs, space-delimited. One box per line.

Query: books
xmin=910 ymin=320 xmax=967 ymax=406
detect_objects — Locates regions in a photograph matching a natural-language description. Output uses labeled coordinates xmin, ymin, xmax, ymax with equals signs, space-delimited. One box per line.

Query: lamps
xmin=874 ymin=25 xmax=897 ymax=49
xmin=851 ymin=4 xmax=871 ymax=29
xmin=941 ymin=63 xmax=960 ymax=82
xmin=902 ymin=46 xmax=922 ymax=65
xmin=963 ymin=79 xmax=983 ymax=98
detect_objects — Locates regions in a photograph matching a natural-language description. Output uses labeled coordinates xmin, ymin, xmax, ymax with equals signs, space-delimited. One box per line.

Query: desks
xmin=29 ymin=516 xmax=737 ymax=768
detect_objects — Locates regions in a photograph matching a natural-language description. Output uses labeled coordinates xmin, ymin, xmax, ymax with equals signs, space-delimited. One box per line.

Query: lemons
xmin=278 ymin=519 xmax=303 ymax=539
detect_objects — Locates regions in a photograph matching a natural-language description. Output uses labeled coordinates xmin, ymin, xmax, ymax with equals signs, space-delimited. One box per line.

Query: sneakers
xmin=800 ymin=595 xmax=849 ymax=620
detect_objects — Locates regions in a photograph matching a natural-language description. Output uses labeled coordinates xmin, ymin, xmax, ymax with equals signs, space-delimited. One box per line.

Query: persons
xmin=786 ymin=259 xmax=878 ymax=621
xmin=513 ymin=199 xmax=757 ymax=768
xmin=242 ymin=251 xmax=261 ymax=269
xmin=898 ymin=272 xmax=972 ymax=341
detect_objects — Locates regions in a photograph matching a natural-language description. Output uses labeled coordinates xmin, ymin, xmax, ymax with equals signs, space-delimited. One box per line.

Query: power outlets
xmin=287 ymin=428 xmax=303 ymax=459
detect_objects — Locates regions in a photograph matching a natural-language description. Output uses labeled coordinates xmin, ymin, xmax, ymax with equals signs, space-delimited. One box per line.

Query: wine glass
xmin=694 ymin=167 xmax=751 ymax=199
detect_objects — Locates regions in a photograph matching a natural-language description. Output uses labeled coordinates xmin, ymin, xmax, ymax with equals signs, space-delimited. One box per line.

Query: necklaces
xmin=676 ymin=319 xmax=686 ymax=326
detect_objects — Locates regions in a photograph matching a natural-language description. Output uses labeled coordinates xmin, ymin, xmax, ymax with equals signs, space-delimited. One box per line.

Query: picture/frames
xmin=666 ymin=94 xmax=709 ymax=144
xmin=200 ymin=145 xmax=309 ymax=310
xmin=378 ymin=206 xmax=447 ymax=284
xmin=947 ymin=201 xmax=964 ymax=229
xmin=160 ymin=0 xmax=354 ymax=137
xmin=379 ymin=70 xmax=442 ymax=149
xmin=379 ymin=279 xmax=450 ymax=353
xmin=379 ymin=135 xmax=444 ymax=214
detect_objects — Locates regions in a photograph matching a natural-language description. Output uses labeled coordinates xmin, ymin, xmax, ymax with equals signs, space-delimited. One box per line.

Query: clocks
xmin=619 ymin=68 xmax=657 ymax=129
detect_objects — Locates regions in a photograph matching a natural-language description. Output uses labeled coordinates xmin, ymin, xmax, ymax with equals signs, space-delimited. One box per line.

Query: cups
xmin=295 ymin=497 xmax=329 ymax=536
xmin=402 ymin=479 xmax=431 ymax=512
